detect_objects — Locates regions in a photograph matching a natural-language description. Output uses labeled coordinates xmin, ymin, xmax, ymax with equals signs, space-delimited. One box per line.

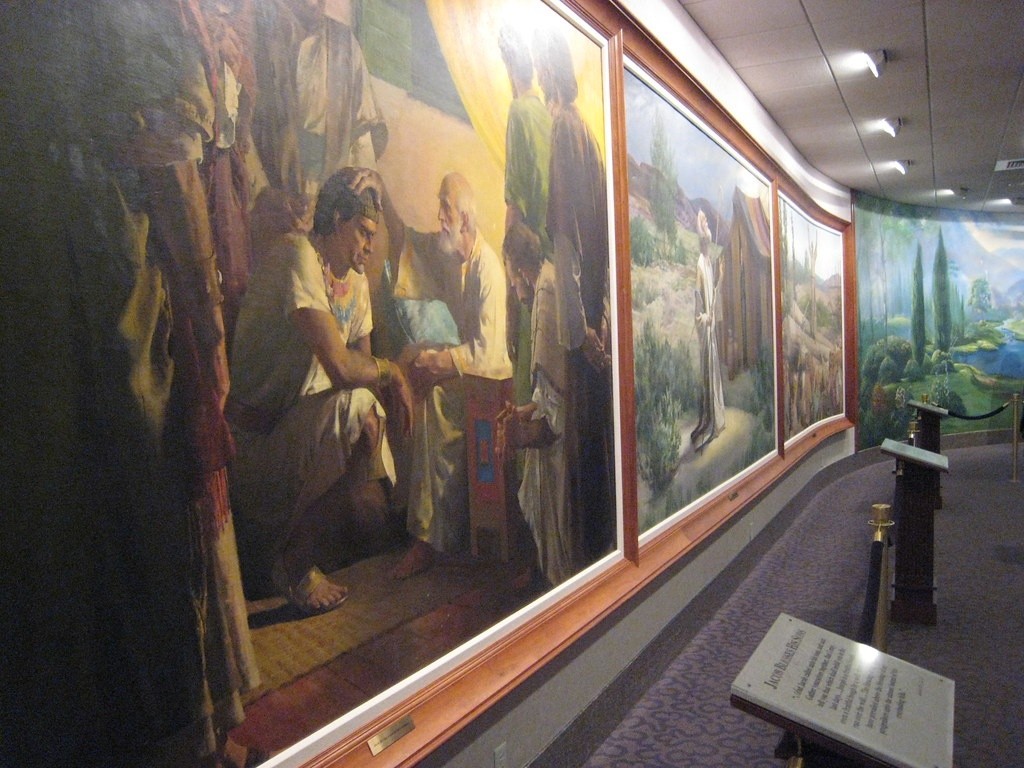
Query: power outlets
xmin=493 ymin=741 xmax=507 ymax=768
xmin=822 ymin=455 xmax=825 ymax=467
xmin=750 ymin=523 xmax=755 ymax=541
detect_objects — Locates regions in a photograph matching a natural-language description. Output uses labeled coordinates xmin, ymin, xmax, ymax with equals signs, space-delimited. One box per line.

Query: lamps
xmin=897 ymin=160 xmax=912 ymax=175
xmin=883 ymin=117 xmax=904 ymax=137
xmin=863 ymin=48 xmax=889 ymax=78
xmin=1008 ymin=193 xmax=1024 ymax=206
